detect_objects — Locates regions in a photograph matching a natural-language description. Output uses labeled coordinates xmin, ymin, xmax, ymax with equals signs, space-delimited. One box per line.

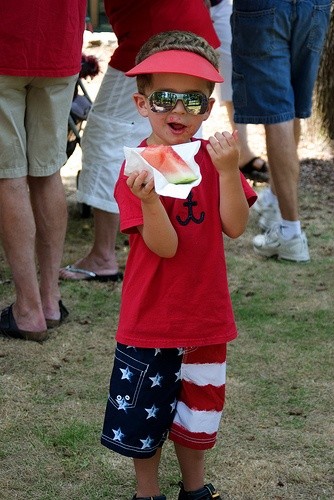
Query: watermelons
xmin=138 ymin=144 xmax=198 ymax=184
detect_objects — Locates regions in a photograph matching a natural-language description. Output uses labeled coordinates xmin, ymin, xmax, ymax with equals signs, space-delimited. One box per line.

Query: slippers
xmin=0 ymin=302 xmax=47 ymax=342
xmin=57 ymin=265 xmax=123 ymax=283
xmin=44 ymin=300 xmax=69 ymax=328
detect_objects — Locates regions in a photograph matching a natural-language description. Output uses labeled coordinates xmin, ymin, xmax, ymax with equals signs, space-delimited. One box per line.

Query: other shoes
xmin=239 ymin=156 xmax=271 ymax=183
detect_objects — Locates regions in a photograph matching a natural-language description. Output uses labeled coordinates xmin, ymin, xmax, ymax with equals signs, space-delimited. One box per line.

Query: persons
xmin=99 ymin=31 xmax=259 ymax=499
xmin=209 ymin=0 xmax=273 ymax=183
xmin=228 ymin=0 xmax=332 ymax=264
xmin=0 ymin=2 xmax=88 ymax=343
xmin=56 ymin=0 xmax=222 ymax=280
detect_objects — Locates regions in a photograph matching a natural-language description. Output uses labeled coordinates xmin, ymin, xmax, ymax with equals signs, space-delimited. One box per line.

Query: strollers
xmin=65 ymin=54 xmax=96 ymax=219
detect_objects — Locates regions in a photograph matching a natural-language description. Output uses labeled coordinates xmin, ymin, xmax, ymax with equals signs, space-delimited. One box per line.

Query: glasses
xmin=145 ymin=91 xmax=209 ymax=115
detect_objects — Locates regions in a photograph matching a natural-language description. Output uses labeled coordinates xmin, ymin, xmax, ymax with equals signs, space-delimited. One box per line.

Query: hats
xmin=125 ymin=45 xmax=225 ymax=83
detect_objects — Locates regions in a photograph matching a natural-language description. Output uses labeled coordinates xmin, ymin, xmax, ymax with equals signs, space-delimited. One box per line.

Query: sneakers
xmin=249 ymin=188 xmax=282 ymax=231
xmin=177 ymin=480 xmax=221 ymax=500
xmin=132 ymin=493 xmax=166 ymax=500
xmin=253 ymin=222 xmax=311 ymax=264
